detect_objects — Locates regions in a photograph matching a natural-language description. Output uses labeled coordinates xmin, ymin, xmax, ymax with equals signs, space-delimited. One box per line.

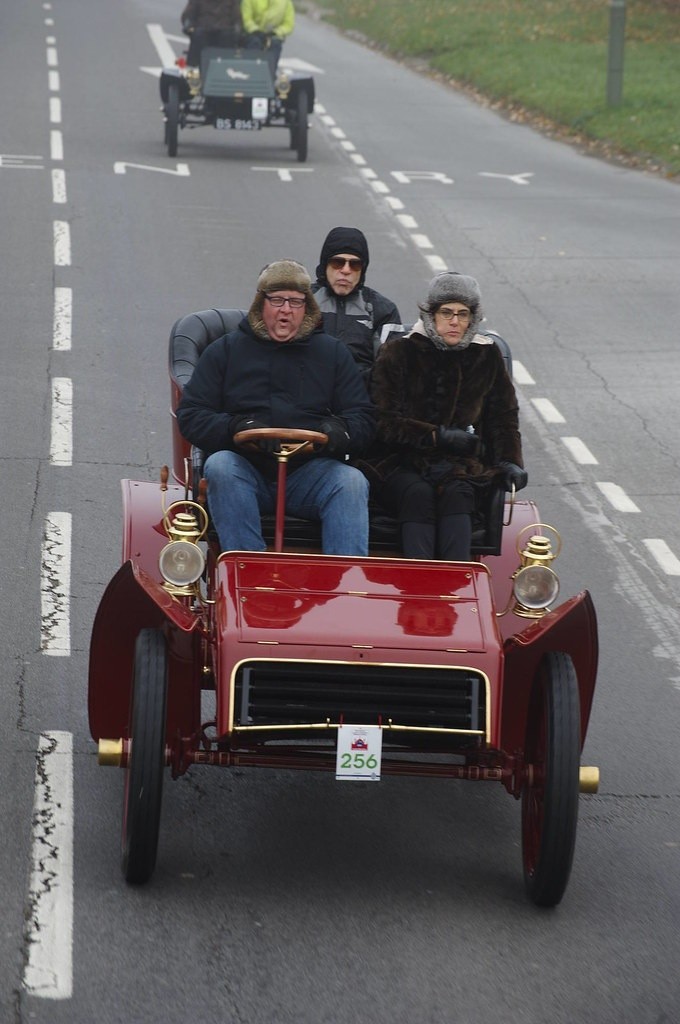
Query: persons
xmin=175 ymin=1 xmax=245 ymax=67
xmin=305 ymin=226 xmax=406 ymax=377
xmin=163 ymin=260 xmax=372 ymax=558
xmin=369 ymin=270 xmax=532 ymax=563
xmin=241 ymin=0 xmax=297 ymax=80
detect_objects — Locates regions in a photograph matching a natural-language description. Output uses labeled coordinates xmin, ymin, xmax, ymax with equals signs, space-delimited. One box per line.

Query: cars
xmin=164 ymin=26 xmax=316 ymax=164
xmin=87 ymin=299 xmax=605 ymax=911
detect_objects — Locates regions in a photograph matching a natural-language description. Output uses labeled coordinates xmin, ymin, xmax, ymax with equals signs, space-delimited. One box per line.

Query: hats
xmin=318 ymin=226 xmax=370 ymax=267
xmin=247 ymin=259 xmax=323 ymax=342
xmin=417 ymin=271 xmax=485 ymax=351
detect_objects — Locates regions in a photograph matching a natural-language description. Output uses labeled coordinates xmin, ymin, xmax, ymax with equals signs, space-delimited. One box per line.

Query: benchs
xmin=166 ymin=302 xmax=520 ymax=557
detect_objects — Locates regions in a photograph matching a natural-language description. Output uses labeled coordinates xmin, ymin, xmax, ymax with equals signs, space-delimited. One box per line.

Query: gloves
xmin=440 ymin=425 xmax=479 ymax=457
xmin=313 ymin=415 xmax=349 ymax=459
xmin=238 ymin=417 xmax=282 ymax=454
xmin=496 ymin=460 xmax=528 ymax=493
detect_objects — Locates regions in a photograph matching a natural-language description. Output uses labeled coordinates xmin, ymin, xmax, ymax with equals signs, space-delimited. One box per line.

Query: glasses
xmin=264 ymin=293 xmax=309 ymax=308
xmin=436 ymin=308 xmax=475 ymax=323
xmin=325 ymin=257 xmax=368 ymax=271
